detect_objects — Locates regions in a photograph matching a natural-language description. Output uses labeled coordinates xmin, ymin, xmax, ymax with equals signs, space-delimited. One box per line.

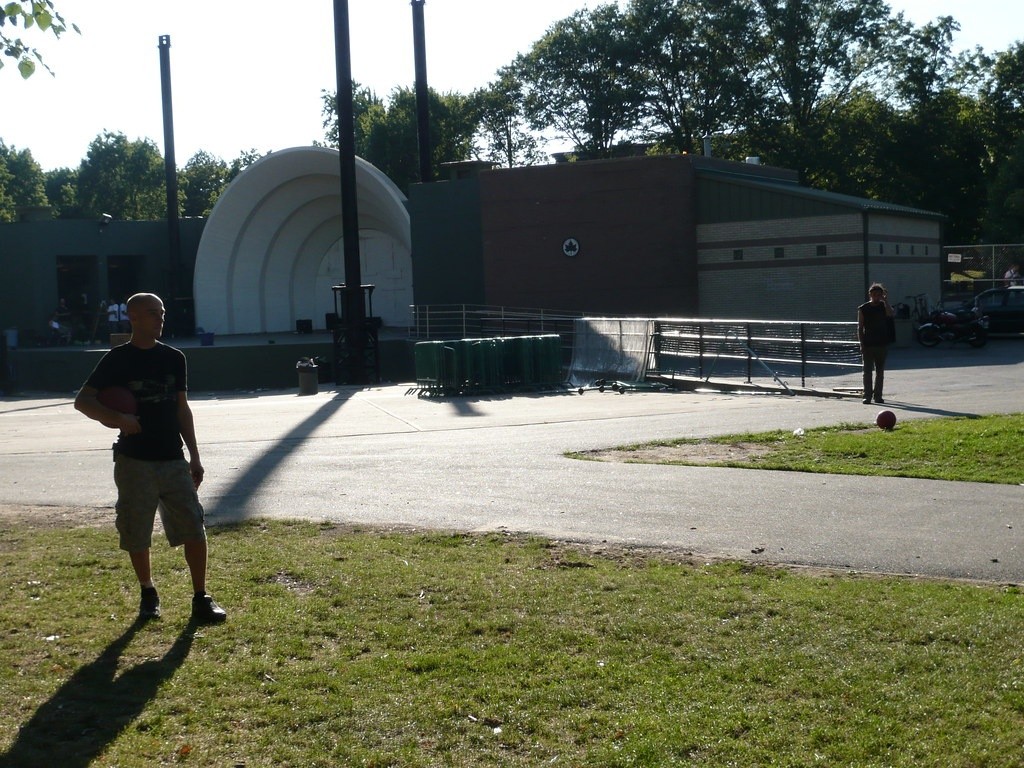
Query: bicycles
xmin=906 ymin=293 xmax=930 ymax=324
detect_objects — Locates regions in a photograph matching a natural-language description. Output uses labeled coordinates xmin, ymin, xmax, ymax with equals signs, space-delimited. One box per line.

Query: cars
xmin=929 ymin=286 xmax=1024 ymax=334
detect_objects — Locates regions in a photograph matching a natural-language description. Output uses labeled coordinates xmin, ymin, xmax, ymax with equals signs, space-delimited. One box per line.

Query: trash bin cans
xmin=296 ymin=363 xmax=319 ymax=394
xmin=894 ymin=319 xmax=913 ymax=348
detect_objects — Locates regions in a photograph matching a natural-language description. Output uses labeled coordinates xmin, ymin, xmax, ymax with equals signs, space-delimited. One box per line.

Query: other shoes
xmin=877 ymin=398 xmax=884 ymax=403
xmin=139 ymin=587 xmax=161 ymax=619
xmin=191 ymin=594 xmax=226 ymax=622
xmin=863 ymin=399 xmax=872 ymax=404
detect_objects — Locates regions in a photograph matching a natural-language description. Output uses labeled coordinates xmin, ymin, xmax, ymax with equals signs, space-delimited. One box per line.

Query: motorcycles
xmin=916 ymin=307 xmax=989 ymax=348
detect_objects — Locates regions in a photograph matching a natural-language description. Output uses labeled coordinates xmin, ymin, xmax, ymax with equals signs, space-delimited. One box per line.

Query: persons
xmin=858 ymin=282 xmax=895 ymax=404
xmin=74 ymin=293 xmax=227 ymax=622
xmin=105 ymin=298 xmax=129 ymax=334
xmin=53 ymin=297 xmax=70 ymax=319
xmin=1003 ymin=265 xmax=1019 ymax=288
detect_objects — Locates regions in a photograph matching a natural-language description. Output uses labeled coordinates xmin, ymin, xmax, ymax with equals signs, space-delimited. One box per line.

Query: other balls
xmin=98 ymin=387 xmax=135 ymax=428
xmin=877 ymin=411 xmax=897 ymax=429
